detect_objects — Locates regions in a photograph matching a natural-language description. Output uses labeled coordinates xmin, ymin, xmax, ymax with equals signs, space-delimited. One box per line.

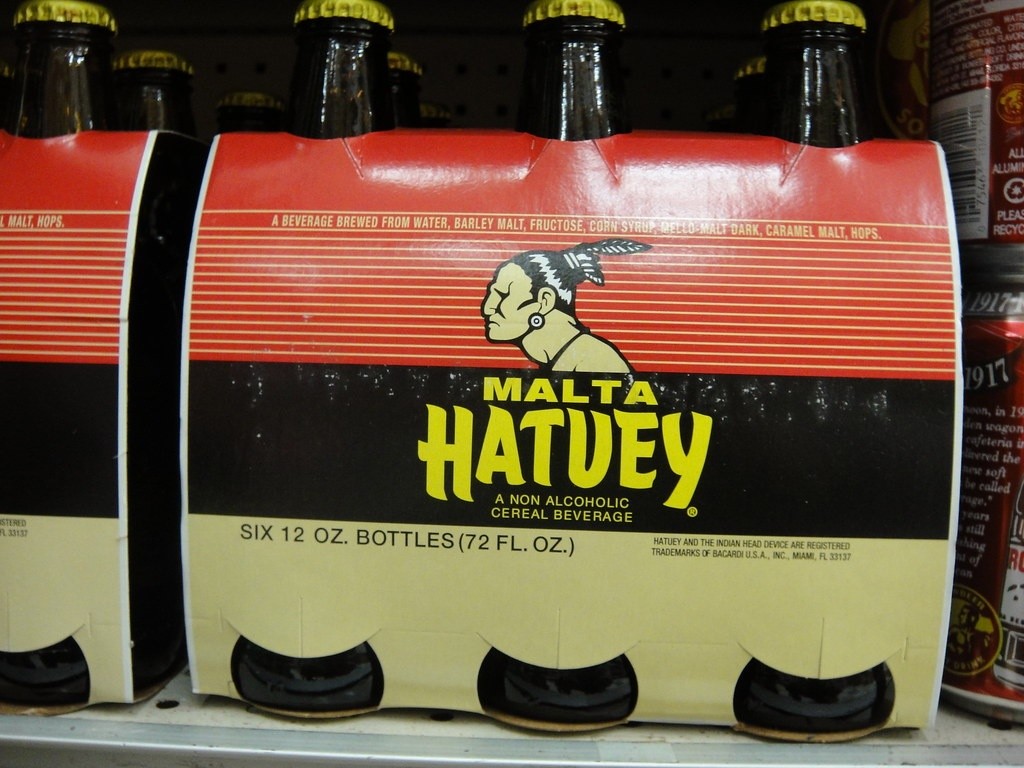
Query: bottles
xmin=0 ymin=0 xmax=898 ymax=745
